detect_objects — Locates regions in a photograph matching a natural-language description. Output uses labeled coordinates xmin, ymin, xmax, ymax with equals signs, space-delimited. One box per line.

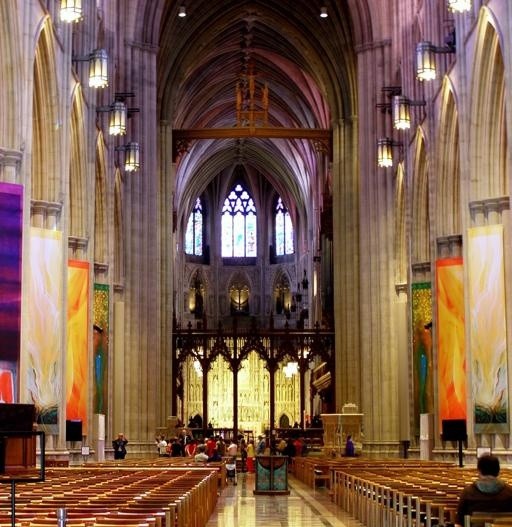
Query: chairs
xmin=288 ymin=449 xmax=512 ymax=527
xmin=0 ymin=457 xmax=233 ymax=525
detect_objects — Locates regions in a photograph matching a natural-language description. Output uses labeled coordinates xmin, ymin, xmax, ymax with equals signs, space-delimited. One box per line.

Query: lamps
xmin=57 ymin=2 xmax=146 ymax=174
xmin=373 ymin=15 xmax=453 ymax=172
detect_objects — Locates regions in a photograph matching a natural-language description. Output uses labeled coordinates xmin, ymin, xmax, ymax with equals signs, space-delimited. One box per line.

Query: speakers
xmin=442 ymin=418 xmax=465 ymax=441
xmin=65 ymin=419 xmax=83 ymax=442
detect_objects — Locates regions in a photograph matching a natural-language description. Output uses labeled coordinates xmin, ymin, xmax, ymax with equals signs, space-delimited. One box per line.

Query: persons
xmin=454 ymin=453 xmax=512 ymax=526
xmin=112 ymin=433 xmax=128 ymax=460
xmin=345 ymin=435 xmax=354 ymax=456
xmin=157 ymin=429 xmax=307 ymax=482
